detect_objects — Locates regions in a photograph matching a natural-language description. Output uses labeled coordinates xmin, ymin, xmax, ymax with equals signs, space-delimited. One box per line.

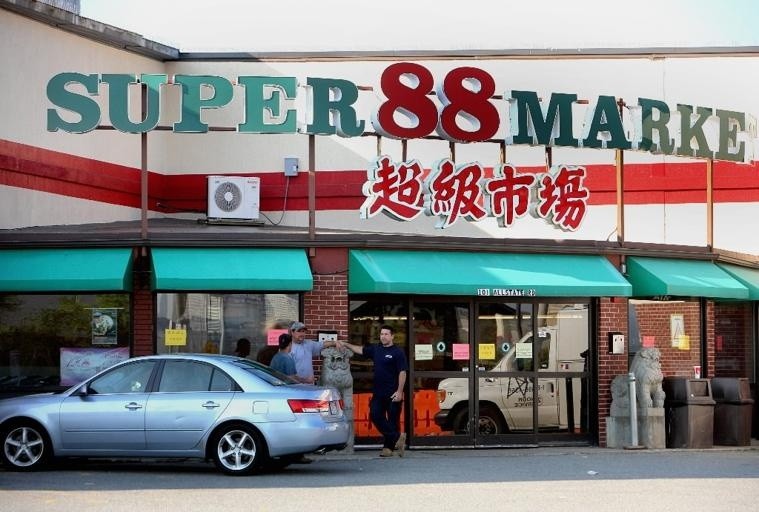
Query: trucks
xmin=435 ymin=309 xmax=589 ymax=435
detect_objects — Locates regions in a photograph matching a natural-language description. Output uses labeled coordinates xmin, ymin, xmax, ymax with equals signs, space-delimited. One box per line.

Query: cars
xmin=0 ymin=353 xmax=350 ymax=476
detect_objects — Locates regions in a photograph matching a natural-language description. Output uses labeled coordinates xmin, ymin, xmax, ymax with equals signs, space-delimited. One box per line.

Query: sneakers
xmin=294 ymin=456 xmax=313 ymax=464
xmin=379 ymin=448 xmax=393 ymax=457
xmin=396 ymin=433 xmax=406 ymax=457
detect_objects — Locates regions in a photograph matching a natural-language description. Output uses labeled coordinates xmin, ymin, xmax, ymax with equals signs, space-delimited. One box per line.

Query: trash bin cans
xmin=663 ymin=376 xmax=753 ymax=447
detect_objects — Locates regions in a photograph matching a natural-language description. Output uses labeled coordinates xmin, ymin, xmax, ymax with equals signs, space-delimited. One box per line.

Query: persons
xmin=234 ymin=338 xmax=251 ymax=357
xmin=288 ymin=322 xmax=345 ymax=387
xmin=341 ymin=325 xmax=408 ymax=457
xmin=270 ymin=334 xmax=312 ymax=464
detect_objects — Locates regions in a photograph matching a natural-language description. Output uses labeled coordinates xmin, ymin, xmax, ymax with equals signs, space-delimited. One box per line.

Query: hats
xmin=278 ymin=334 xmax=292 ymax=349
xmin=291 ymin=322 xmax=307 ymax=331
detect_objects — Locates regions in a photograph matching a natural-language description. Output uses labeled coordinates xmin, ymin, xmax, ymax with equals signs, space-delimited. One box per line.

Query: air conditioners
xmin=207 ymin=175 xmax=261 ymax=221
